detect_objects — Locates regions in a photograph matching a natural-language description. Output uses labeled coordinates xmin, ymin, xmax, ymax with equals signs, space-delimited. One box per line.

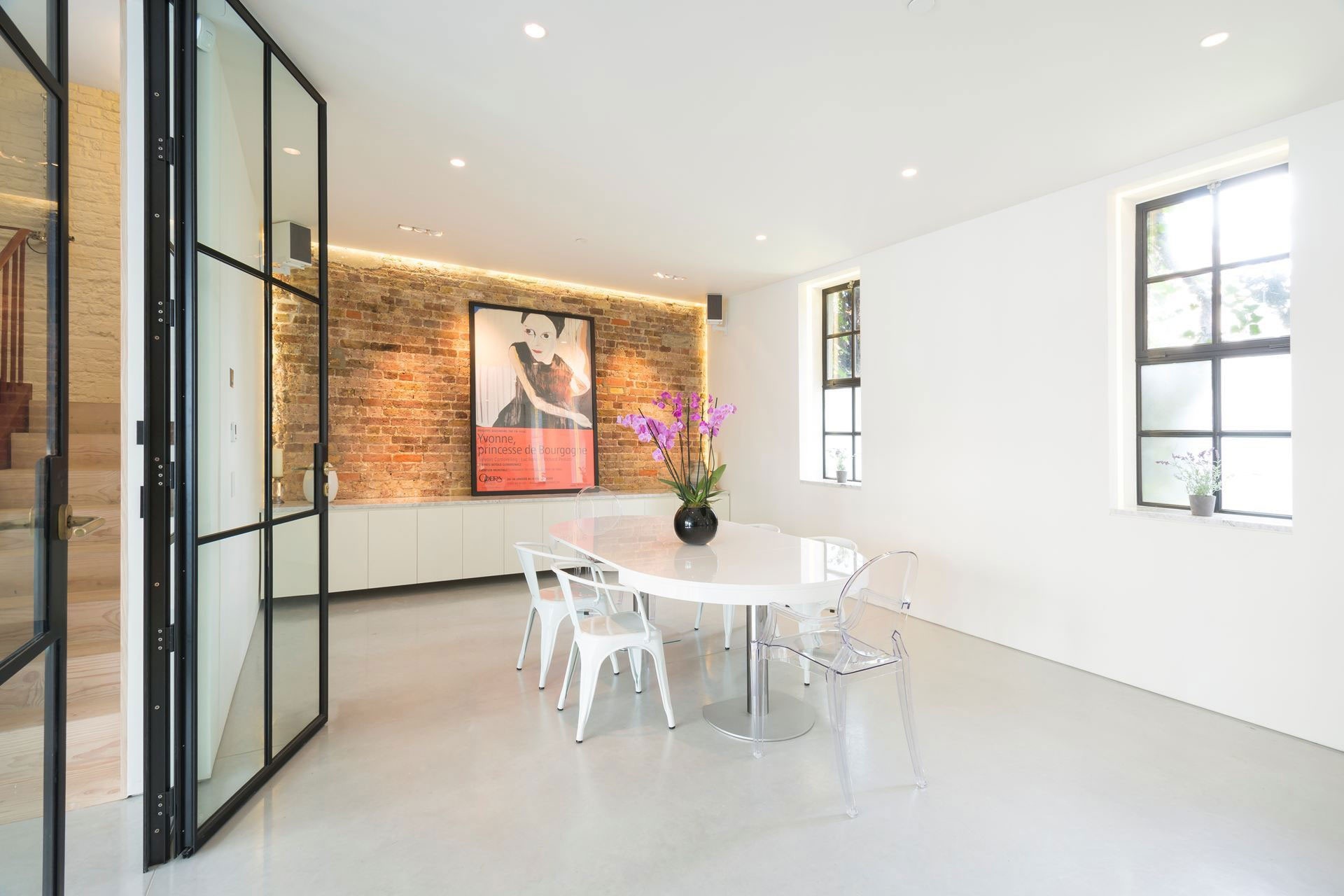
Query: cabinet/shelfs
xmin=265 ymin=492 xmax=747 ymax=603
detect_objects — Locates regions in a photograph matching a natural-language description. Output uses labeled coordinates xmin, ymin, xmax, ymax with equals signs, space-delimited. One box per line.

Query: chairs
xmin=736 ymin=516 xmax=784 ymax=537
xmin=513 ymin=538 xmax=616 ymax=698
xmin=772 ymin=602 xmax=830 ymax=693
xmin=578 ymin=484 xmax=621 ymax=525
xmin=691 ymin=596 xmax=739 ymax=659
xmin=759 ymin=547 xmax=930 ymax=818
xmin=546 ymin=556 xmax=678 ymax=748
xmin=804 ymin=533 xmax=860 ymax=558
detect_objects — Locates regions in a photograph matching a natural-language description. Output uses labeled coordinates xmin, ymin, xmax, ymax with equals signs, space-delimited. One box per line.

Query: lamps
xmin=271 ymin=447 xmax=285 ymax=504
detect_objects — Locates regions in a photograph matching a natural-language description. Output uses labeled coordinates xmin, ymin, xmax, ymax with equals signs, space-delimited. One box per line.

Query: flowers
xmin=1154 ymin=447 xmax=1230 ymax=496
xmin=828 ymin=444 xmax=855 ymax=471
xmin=619 ymin=384 xmax=742 ymax=510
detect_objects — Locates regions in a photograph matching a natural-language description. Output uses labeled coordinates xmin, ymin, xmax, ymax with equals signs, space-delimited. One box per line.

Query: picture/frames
xmin=460 ymin=294 xmax=605 ymax=496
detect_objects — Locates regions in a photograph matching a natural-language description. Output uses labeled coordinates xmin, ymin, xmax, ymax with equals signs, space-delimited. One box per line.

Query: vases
xmin=299 ymin=457 xmax=339 ymax=503
xmin=670 ymin=500 xmax=720 ymax=546
xmin=835 ymin=470 xmax=847 ymax=485
xmin=1185 ymin=492 xmax=1220 ymax=519
xmin=688 ymin=456 xmax=710 ymax=489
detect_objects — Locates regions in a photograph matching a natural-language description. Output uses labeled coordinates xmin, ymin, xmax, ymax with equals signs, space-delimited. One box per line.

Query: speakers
xmin=273 ymin=220 xmax=312 ymax=268
xmin=706 ymin=294 xmax=723 ymax=320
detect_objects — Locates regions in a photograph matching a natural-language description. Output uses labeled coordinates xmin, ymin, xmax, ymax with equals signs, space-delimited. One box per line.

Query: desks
xmin=547 ymin=514 xmax=867 ymax=747
